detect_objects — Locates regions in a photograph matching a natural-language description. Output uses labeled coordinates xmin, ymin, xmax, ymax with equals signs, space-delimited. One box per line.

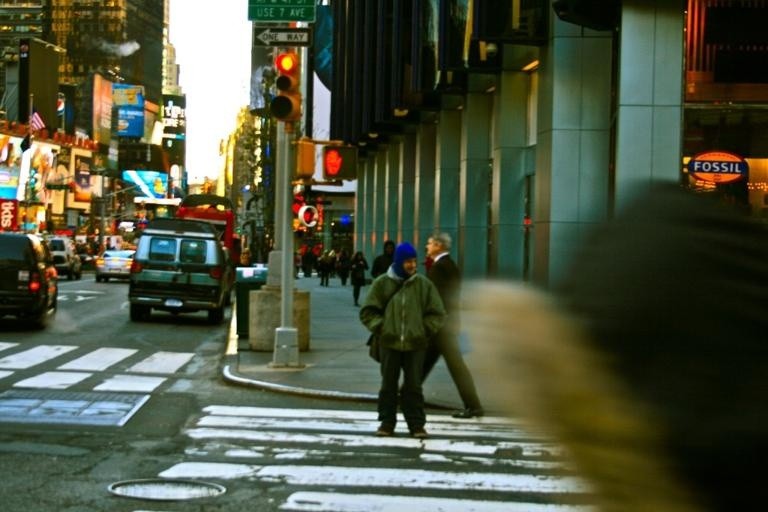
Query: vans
xmin=129 ymin=217 xmax=232 ymax=324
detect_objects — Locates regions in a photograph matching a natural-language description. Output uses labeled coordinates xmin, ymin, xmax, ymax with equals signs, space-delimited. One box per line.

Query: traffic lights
xmin=269 ymin=51 xmax=301 ymax=123
xmin=324 ymin=145 xmax=357 ymax=179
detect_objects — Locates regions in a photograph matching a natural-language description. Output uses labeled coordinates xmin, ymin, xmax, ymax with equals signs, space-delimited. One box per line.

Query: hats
xmin=393 ymin=242 xmax=418 ymax=277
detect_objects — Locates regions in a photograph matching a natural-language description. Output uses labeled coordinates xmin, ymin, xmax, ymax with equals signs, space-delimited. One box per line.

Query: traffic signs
xmin=252 ymin=27 xmax=313 ymax=47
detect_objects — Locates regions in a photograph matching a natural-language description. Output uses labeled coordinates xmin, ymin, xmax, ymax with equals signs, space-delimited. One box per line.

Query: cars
xmin=95 ymin=249 xmax=137 ymax=282
xmin=0 ymin=233 xmax=83 ymax=330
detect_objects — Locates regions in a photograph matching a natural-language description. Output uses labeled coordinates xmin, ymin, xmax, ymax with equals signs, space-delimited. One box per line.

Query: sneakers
xmin=376 ymin=426 xmax=395 ymax=438
xmin=410 ymin=429 xmax=429 ymax=439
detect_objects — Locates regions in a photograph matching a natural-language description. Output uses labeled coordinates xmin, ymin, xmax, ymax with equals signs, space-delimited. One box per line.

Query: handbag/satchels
xmin=366 ymin=333 xmax=381 ymax=363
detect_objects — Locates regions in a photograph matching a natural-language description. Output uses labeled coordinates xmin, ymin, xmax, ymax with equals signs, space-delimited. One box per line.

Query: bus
xmin=175 ymin=194 xmax=235 ymax=257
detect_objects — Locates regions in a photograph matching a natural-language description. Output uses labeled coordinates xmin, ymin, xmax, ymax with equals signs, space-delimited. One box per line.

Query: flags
xmin=31 ymin=109 xmax=47 ymax=134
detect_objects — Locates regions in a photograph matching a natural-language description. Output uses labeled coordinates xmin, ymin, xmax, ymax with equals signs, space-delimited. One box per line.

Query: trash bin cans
xmin=235 ymin=263 xmax=269 ymax=339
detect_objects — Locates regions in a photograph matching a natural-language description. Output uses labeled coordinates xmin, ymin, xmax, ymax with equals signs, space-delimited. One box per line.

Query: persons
xmin=458 ymin=178 xmax=766 ymax=511
xmin=417 ymin=230 xmax=485 ymax=418
xmin=357 ymin=240 xmax=446 ymax=437
xmin=302 ymin=240 xmax=398 ymax=305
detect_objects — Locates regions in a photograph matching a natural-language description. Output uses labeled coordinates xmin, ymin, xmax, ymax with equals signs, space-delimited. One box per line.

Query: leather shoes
xmin=452 ymin=407 xmax=486 ymax=419
